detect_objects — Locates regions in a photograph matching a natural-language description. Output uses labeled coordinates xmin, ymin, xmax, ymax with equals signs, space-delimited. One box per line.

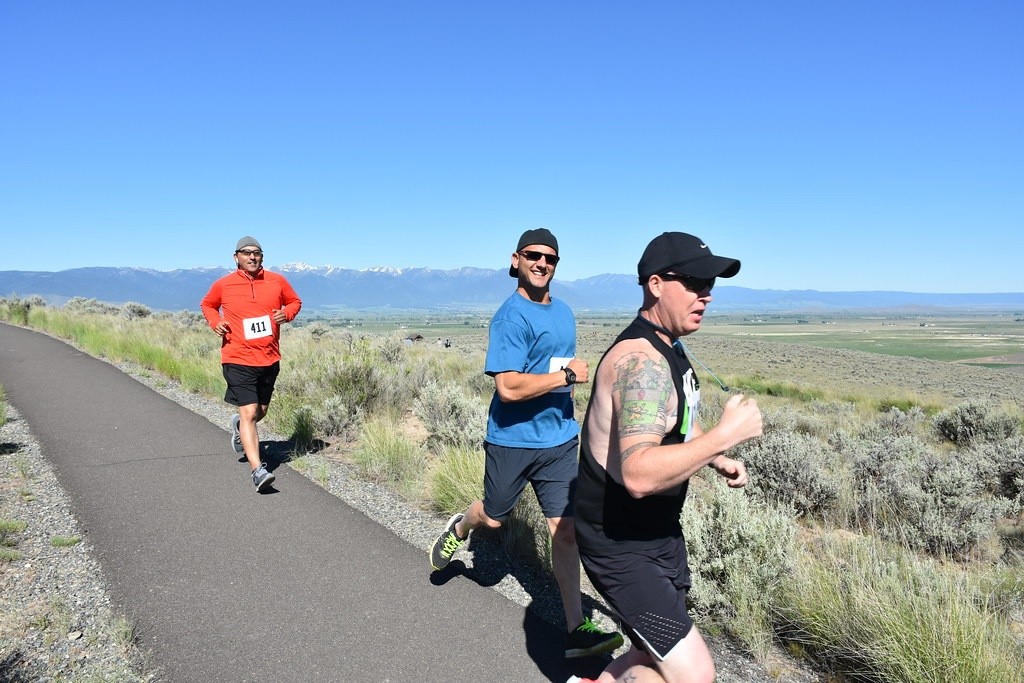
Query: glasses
xmin=660 ymin=274 xmax=715 ymax=291
xmin=519 ymin=251 xmax=560 ymax=264
xmin=235 ymin=249 xmax=262 ymax=257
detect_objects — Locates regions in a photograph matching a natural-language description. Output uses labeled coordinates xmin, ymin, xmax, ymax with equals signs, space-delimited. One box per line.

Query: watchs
xmin=561 ymin=366 xmax=576 ymax=387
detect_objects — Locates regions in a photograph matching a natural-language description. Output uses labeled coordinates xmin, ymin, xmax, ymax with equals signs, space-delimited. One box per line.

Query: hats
xmin=509 ymin=228 xmax=558 ymax=278
xmin=235 ymin=236 xmax=261 ymax=251
xmin=638 ymin=232 xmax=740 ymax=280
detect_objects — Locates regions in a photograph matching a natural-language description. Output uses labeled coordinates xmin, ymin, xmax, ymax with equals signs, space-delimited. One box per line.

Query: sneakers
xmin=251 ymin=459 xmax=275 ymax=492
xmin=564 ymin=617 xmax=624 ymax=659
xmin=430 ymin=512 xmax=469 ymax=570
xmin=231 ymin=415 xmax=244 ymax=453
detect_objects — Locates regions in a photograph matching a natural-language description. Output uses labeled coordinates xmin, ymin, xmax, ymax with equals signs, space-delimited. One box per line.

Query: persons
xmin=429 ymin=228 xmax=624 ymax=660
xmin=200 ymin=235 xmax=302 ymax=491
xmin=565 ymin=233 xmax=763 ymax=683
